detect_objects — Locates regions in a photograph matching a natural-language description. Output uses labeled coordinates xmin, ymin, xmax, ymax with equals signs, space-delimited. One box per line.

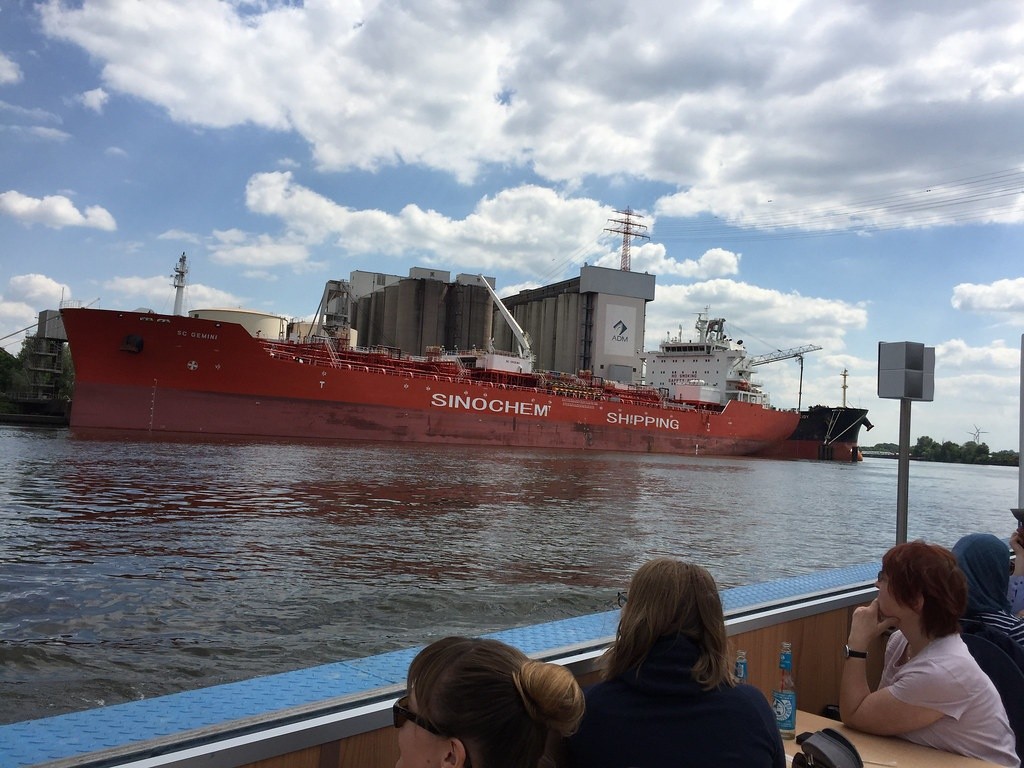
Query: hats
xmin=1009 ymin=507 xmax=1024 ymax=525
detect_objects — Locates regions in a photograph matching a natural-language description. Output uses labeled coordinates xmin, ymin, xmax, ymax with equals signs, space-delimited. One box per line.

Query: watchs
xmin=843 ymin=645 xmax=868 ymax=658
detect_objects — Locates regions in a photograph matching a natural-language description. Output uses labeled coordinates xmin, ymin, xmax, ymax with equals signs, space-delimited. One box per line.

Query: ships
xmin=55 ymin=205 xmax=874 ymax=462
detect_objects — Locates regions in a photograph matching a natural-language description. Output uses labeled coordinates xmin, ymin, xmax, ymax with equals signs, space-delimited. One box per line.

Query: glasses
xmin=616 ymin=589 xmax=628 ymax=605
xmin=1006 ymin=558 xmax=1015 ymax=573
xmin=876 ymin=569 xmax=885 ymax=580
xmin=390 ymin=695 xmax=445 ymax=736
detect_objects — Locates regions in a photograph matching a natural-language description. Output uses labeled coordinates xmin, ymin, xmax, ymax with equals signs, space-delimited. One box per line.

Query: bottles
xmin=734 ymin=650 xmax=747 ymax=685
xmin=771 ymin=641 xmax=796 ymax=741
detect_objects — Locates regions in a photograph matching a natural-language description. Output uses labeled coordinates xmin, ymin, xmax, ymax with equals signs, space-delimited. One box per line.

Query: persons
xmin=838 ymin=539 xmax=1021 ymax=768
xmin=550 ymin=558 xmax=787 ymax=768
xmin=951 ymin=528 xmax=1024 ymax=647
xmin=394 ymin=636 xmax=586 ymax=768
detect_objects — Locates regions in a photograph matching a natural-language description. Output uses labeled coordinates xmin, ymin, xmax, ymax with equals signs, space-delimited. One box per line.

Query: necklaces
xmin=905 ymin=656 xmax=912 ymax=660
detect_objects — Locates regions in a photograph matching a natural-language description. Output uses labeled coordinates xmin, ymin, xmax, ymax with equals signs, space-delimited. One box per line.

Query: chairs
xmin=959 ymin=618 xmax=1024 ymax=768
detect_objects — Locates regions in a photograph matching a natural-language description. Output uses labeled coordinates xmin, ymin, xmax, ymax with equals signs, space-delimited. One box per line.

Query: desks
xmin=772 ymin=705 xmax=1011 ymax=768
